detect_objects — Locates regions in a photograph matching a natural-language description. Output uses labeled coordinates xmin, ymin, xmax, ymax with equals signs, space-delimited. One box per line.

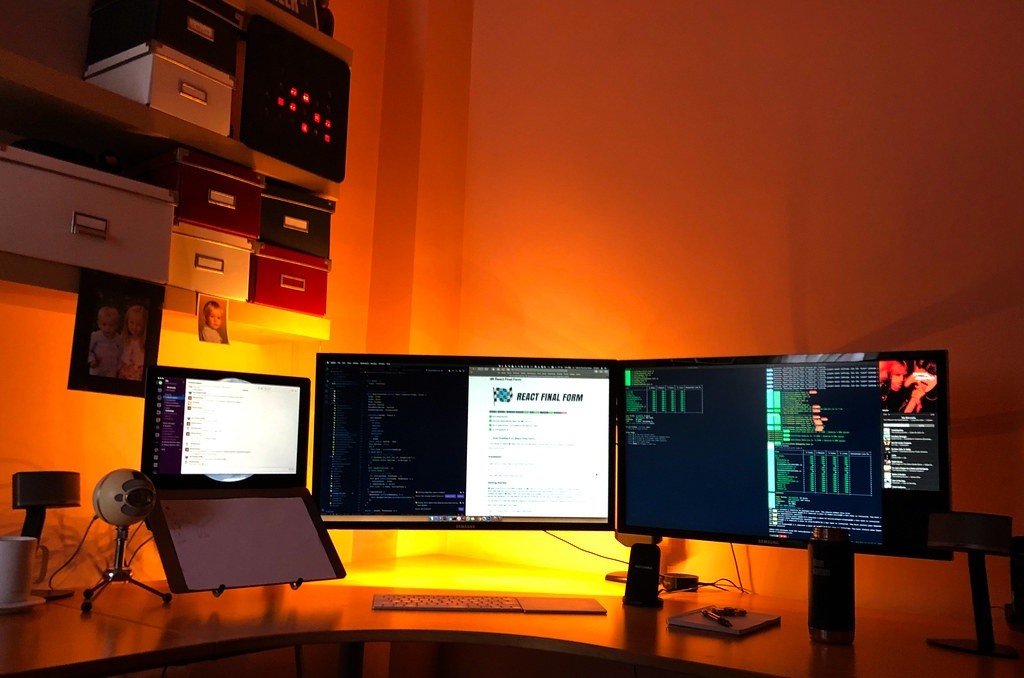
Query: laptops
xmin=140 ymin=367 xmax=346 ymax=594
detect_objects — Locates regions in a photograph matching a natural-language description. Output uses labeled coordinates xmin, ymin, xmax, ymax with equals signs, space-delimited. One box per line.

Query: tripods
xmin=80 ymin=526 xmax=171 ymax=613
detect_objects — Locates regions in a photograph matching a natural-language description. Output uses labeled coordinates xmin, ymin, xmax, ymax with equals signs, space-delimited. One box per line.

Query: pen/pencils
xmin=701 ymin=609 xmax=732 ymax=627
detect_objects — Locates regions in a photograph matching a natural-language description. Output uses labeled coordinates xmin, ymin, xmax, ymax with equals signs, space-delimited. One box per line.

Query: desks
xmin=1 ymin=551 xmax=1024 ymax=678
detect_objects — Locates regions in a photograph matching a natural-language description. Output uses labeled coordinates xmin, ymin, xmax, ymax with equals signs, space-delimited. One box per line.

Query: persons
xmin=879 ymin=359 xmax=937 ymax=414
xmin=87 ymin=306 xmax=148 ymax=381
xmin=201 ymin=301 xmax=223 ymax=344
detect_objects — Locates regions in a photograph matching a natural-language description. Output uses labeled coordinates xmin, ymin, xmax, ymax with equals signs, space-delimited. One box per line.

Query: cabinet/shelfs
xmin=0 ymin=0 xmax=354 ymax=342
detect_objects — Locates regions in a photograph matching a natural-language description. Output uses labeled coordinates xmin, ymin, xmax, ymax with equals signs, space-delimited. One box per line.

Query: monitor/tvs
xmin=314 ymin=349 xmax=956 ymax=564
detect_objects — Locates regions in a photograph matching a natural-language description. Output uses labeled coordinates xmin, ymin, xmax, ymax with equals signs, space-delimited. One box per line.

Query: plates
xmin=0 ymin=594 xmax=47 ymax=614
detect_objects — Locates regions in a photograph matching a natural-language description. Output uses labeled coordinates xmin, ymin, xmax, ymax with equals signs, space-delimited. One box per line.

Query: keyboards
xmin=372 ymin=594 xmax=523 ymax=612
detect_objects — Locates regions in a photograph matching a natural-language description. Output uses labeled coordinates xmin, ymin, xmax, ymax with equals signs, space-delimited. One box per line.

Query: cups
xmin=806 ymin=527 xmax=855 ymax=645
xmin=0 ymin=536 xmax=49 ymax=603
xmin=1004 ymin=535 xmax=1024 ymax=632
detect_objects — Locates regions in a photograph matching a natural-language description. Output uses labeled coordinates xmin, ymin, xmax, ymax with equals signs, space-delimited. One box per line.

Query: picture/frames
xmin=65 ymin=279 xmax=164 ymax=397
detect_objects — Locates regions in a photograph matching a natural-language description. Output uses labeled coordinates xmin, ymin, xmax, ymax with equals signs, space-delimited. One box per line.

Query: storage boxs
xmin=0 ymin=0 xmax=355 ymax=317
